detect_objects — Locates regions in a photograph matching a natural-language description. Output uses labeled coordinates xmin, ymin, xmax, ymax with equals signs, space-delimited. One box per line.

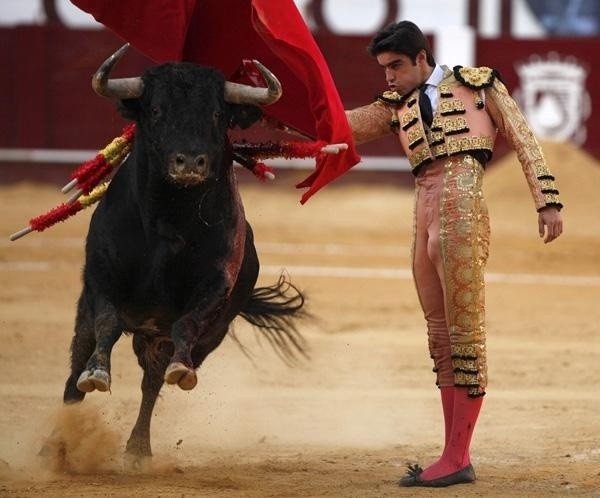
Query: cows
xmin=36 ymin=39 xmax=320 ymax=476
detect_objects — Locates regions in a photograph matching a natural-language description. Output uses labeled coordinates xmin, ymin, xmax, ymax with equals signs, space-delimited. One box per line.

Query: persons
xmin=258 ymin=19 xmax=565 ymax=488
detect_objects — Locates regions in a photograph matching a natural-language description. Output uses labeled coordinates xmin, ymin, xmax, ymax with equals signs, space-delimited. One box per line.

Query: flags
xmin=70 ymin=1 xmax=363 ymax=207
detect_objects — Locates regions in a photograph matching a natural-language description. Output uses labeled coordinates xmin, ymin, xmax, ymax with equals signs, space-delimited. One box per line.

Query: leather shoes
xmin=397 ymin=462 xmax=477 ymax=490
